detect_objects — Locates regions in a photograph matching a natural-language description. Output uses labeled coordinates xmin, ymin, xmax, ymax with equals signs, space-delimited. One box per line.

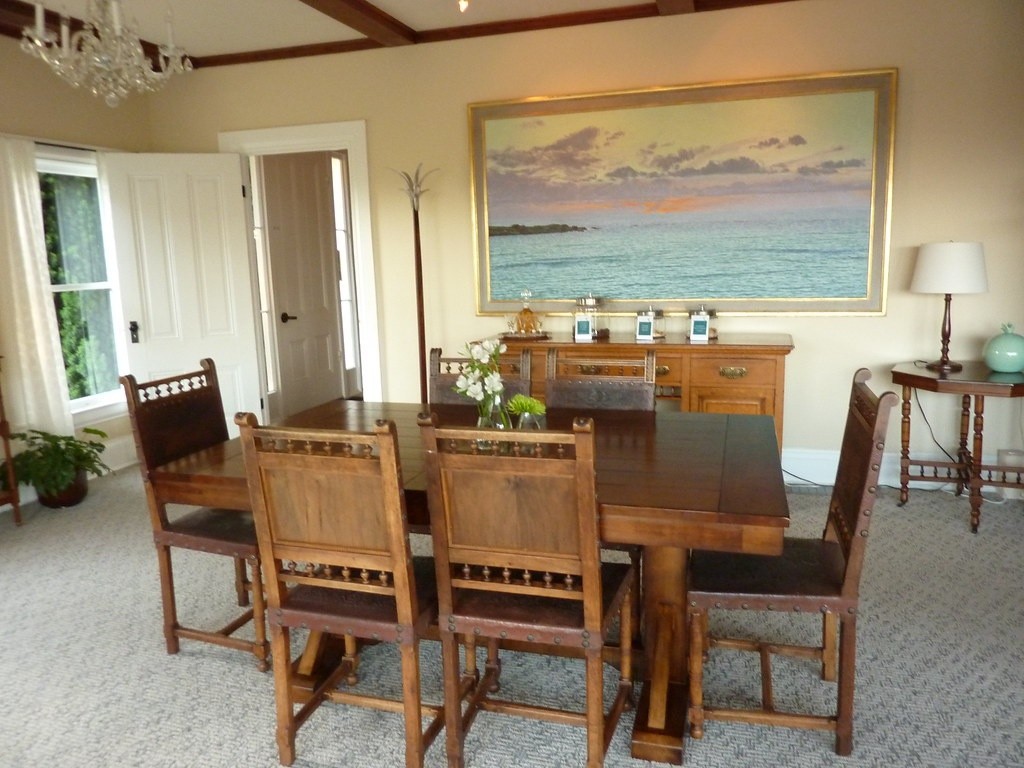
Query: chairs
xmin=418 ymin=412 xmax=635 ymax=768
xmin=690 ymin=367 xmax=901 ymax=756
xmin=233 ymin=410 xmax=477 ymax=768
xmin=426 ymin=346 xmax=659 ymax=411
xmin=119 ymin=358 xmax=272 ymax=673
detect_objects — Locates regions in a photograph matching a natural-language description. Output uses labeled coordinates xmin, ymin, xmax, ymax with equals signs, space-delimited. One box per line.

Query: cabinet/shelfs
xmin=470 ymin=331 xmax=796 ymax=465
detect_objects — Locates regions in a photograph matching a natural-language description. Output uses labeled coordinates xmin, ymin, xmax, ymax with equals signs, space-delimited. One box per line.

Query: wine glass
xmin=504 ymin=313 xmax=546 ymax=337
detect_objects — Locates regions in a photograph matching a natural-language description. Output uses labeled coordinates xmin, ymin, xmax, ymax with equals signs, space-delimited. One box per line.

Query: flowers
xmin=449 ymin=338 xmax=508 ymax=429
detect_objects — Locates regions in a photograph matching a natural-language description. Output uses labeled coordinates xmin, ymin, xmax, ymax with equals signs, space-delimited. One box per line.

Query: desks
xmin=891 ymin=359 xmax=1024 ymax=534
xmin=148 ymin=398 xmax=790 ymax=767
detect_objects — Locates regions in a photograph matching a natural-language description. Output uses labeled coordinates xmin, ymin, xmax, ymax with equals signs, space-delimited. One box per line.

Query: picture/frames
xmin=465 ymin=64 xmax=899 ymax=316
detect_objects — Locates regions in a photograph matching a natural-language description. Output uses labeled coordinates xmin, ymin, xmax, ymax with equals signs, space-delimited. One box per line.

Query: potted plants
xmin=2 ymin=427 xmax=119 ymax=509
xmin=504 ymin=392 xmax=547 ymax=432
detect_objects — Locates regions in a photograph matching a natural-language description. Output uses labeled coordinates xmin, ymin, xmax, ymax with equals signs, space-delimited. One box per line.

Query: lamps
xmin=19 ymin=0 xmax=194 ymax=109
xmin=909 ymin=238 xmax=988 ymax=372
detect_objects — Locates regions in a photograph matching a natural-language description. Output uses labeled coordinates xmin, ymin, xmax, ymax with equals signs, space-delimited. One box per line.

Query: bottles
xmin=572 ymin=292 xmax=610 ymax=339
xmin=634 ymin=305 xmax=666 ymax=339
xmin=513 ymin=289 xmax=540 ymax=332
xmin=686 ymin=303 xmax=719 ymax=340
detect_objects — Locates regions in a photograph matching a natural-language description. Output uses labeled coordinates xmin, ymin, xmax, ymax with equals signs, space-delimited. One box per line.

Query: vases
xmin=472 ymin=391 xmax=512 ymax=431
xmin=981 ymin=321 xmax=1024 ymax=373
